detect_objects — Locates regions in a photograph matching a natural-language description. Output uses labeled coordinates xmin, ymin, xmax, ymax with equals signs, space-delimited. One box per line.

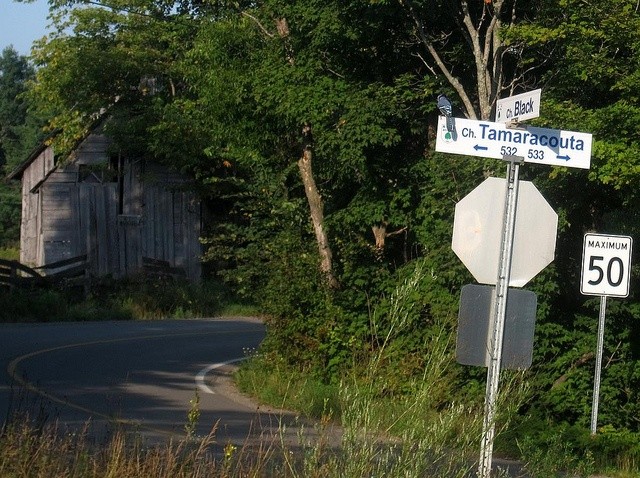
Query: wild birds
xmin=435 ymin=91 xmax=456 ymax=133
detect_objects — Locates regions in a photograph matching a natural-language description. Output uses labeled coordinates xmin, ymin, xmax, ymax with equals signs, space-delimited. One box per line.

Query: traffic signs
xmin=435 ymin=114 xmax=593 ymax=169
xmin=581 ymin=233 xmax=633 ymax=297
xmin=494 ymin=87 xmax=542 ymax=123
